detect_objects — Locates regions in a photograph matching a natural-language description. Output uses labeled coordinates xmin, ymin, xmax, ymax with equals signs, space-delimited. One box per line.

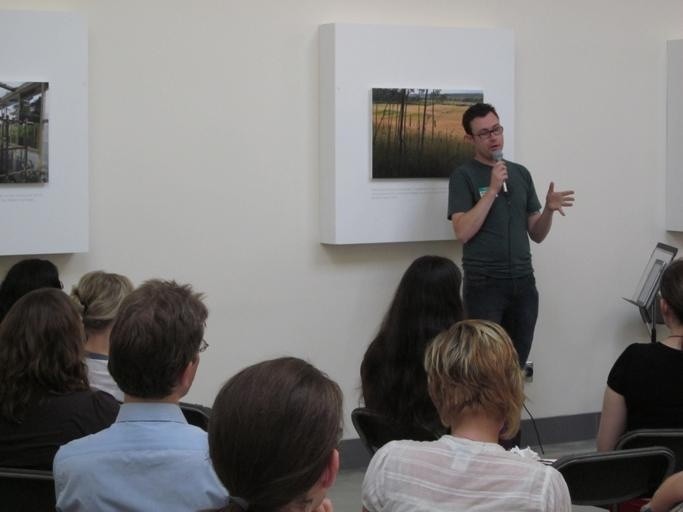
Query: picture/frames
xmin=314 ymin=19 xmax=515 ymax=244
xmin=0 ymin=6 xmax=91 ymax=258
xmin=665 ymin=37 xmax=683 ymax=236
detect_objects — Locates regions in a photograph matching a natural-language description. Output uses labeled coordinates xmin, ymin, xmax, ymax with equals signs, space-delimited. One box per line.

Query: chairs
xmin=178 ymin=402 xmax=213 ymax=430
xmin=547 ymin=443 xmax=674 ymax=512
xmin=1 ymin=469 xmax=56 ymax=512
xmin=616 ymin=431 xmax=683 ymax=470
xmin=351 ymin=408 xmax=399 ymax=455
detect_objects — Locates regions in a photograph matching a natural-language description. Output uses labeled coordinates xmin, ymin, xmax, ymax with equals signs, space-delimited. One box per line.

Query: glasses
xmin=200 ymin=338 xmax=209 ymax=352
xmin=472 ymin=126 xmax=503 ymax=140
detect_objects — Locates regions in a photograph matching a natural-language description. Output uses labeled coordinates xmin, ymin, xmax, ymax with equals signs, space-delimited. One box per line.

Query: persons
xmin=208 ymin=356 xmax=343 ymax=512
xmin=73 ymin=273 xmax=133 ymax=405
xmin=362 ymin=256 xmax=463 ymax=440
xmin=597 ymin=260 xmax=683 ymax=469
xmin=1 ymin=287 xmax=119 ymax=469
xmin=449 ymin=105 xmax=576 ymax=373
xmin=55 ymin=281 xmax=225 ymax=511
xmin=360 ymin=324 xmax=570 ymax=512
xmin=2 ymin=257 xmax=61 ymax=312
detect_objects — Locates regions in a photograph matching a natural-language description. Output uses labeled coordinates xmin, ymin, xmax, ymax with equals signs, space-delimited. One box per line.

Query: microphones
xmin=491 ymin=150 xmax=508 ymax=193
